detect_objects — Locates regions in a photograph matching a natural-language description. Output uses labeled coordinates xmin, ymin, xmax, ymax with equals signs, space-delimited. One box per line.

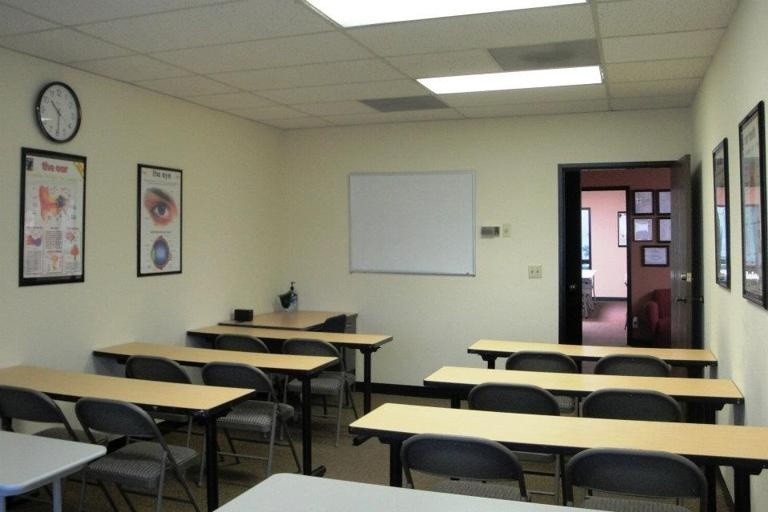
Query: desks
xmin=205 ymin=469 xmax=612 ymax=512
xmin=216 ymin=305 xmax=361 ymax=387
xmin=420 ymin=363 xmax=747 ymax=431
xmin=0 ymin=362 xmax=258 ymax=512
xmin=0 ymin=426 xmax=109 ymax=511
xmin=466 ymin=338 xmax=719 ymax=384
xmin=346 ymin=401 xmax=768 ymax=512
xmin=186 ymin=322 xmax=394 ymax=447
xmin=92 ymin=339 xmax=342 ymax=479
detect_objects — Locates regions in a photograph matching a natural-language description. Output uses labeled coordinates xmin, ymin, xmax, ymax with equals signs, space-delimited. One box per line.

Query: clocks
xmin=34 ymin=80 xmax=83 ymax=144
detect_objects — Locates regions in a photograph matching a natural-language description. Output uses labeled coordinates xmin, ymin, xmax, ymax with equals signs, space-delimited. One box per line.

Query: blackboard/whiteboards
xmin=347 ymin=170 xmax=477 ymax=277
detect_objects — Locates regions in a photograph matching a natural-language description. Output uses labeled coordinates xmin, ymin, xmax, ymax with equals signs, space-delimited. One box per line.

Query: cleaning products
xmin=284 ymin=281 xmax=298 ymax=312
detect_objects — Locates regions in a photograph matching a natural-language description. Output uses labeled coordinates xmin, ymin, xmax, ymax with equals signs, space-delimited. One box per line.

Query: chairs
xmin=277 ymin=336 xmax=362 ymax=451
xmin=556 ymin=446 xmax=709 ymax=512
xmin=579 ymin=267 xmax=598 ymax=319
xmin=503 ymin=348 xmax=581 ymax=375
xmin=320 ymin=311 xmax=350 ymax=416
xmin=398 ymin=429 xmax=538 ymax=506
xmin=468 ymin=376 xmax=562 ymax=501
xmin=72 ymin=395 xmax=207 ymax=512
xmin=0 ymin=382 xmax=129 ymax=512
xmin=212 ymin=330 xmax=296 ymax=439
xmin=591 ymin=350 xmax=676 ymax=379
xmin=643 ymin=286 xmax=671 ymax=348
xmin=123 ymin=351 xmax=225 ymax=481
xmin=199 ymin=357 xmax=303 ymax=487
xmin=577 ymin=388 xmax=683 ymax=504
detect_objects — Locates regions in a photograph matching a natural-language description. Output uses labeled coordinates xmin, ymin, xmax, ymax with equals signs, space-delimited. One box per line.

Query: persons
xmin=142 ymin=186 xmax=179 ymax=228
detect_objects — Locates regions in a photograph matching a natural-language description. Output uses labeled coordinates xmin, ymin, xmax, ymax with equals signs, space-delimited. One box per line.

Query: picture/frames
xmin=135 ymin=162 xmax=184 ymax=279
xmin=709 ymin=135 xmax=732 ymax=293
xmin=654 ymin=217 xmax=673 ymax=244
xmin=734 ymin=100 xmax=768 ymax=309
xmin=616 ymin=210 xmax=627 ymax=247
xmin=16 ymin=146 xmax=87 ymax=288
xmin=656 ymin=188 xmax=673 ymax=215
xmin=632 ymin=188 xmax=656 ymax=216
xmin=640 ymin=244 xmax=669 ymax=267
xmin=631 ymin=217 xmax=654 ymax=242
